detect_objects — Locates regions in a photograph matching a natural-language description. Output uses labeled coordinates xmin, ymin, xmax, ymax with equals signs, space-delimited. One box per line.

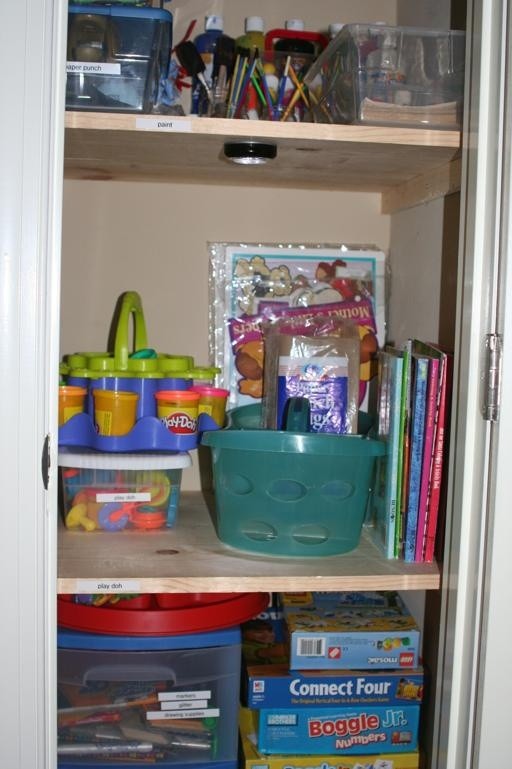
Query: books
xmin=369 ymin=337 xmax=453 ymax=563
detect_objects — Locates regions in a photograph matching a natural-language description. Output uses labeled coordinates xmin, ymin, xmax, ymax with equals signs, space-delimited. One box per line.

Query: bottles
xmin=188 ymin=17 xmax=388 ymax=121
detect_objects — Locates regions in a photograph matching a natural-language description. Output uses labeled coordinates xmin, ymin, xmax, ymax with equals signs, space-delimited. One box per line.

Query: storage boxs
xmin=59 ymin=626 xmax=244 ymax=769
xmin=302 ymin=22 xmax=466 ymax=132
xmin=65 ymin=4 xmax=173 ymax=113
xmin=58 ymin=444 xmax=193 ymax=533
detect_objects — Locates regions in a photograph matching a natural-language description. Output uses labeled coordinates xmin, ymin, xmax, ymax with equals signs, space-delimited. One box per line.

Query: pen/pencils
xmin=226 ymin=46 xmax=336 ymax=123
xmin=59 ymin=733 xmax=212 ymax=753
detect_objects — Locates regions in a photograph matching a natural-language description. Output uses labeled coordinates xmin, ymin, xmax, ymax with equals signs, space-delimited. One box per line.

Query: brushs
xmin=176 ymin=37 xmax=233 ymax=113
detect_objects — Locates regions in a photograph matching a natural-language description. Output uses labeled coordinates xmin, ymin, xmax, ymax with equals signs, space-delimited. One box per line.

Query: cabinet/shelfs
xmin=0 ymin=0 xmax=511 ymax=769
